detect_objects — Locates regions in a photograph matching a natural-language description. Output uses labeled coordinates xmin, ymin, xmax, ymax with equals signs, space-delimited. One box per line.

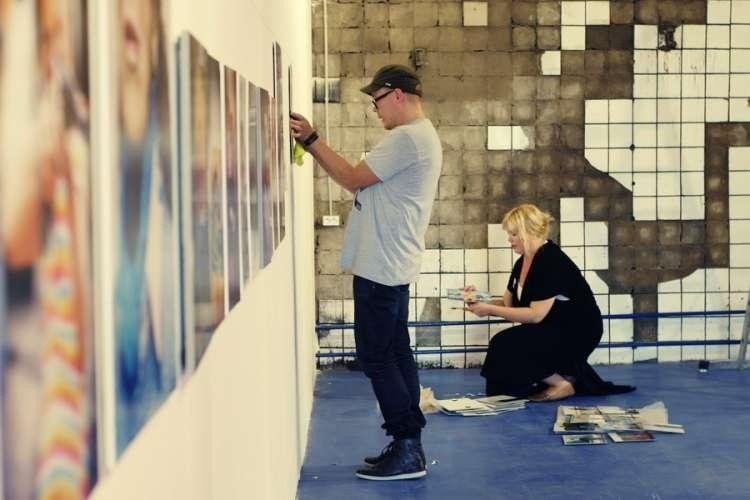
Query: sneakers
xmin=355 ymin=432 xmax=427 ymax=481
xmin=528 ymin=379 xmax=574 ymax=402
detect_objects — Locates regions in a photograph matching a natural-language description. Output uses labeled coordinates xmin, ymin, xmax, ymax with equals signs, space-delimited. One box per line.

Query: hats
xmin=360 ymin=64 xmax=424 ymax=97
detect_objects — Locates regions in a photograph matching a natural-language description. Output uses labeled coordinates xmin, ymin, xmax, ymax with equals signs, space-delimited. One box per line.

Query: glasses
xmin=372 ymin=89 xmax=394 ymax=108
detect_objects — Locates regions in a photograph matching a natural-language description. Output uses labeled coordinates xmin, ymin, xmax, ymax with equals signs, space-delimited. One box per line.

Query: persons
xmin=456 ymin=203 xmax=638 ymax=405
xmin=287 ymin=64 xmax=443 ymax=481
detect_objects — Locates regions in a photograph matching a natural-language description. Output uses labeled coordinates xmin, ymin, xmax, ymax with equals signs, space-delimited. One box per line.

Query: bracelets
xmin=304 ymin=131 xmax=319 ymax=146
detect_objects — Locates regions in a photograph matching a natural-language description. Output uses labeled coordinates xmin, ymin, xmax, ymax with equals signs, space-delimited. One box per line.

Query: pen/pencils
xmin=450 ymin=307 xmax=467 ymax=310
